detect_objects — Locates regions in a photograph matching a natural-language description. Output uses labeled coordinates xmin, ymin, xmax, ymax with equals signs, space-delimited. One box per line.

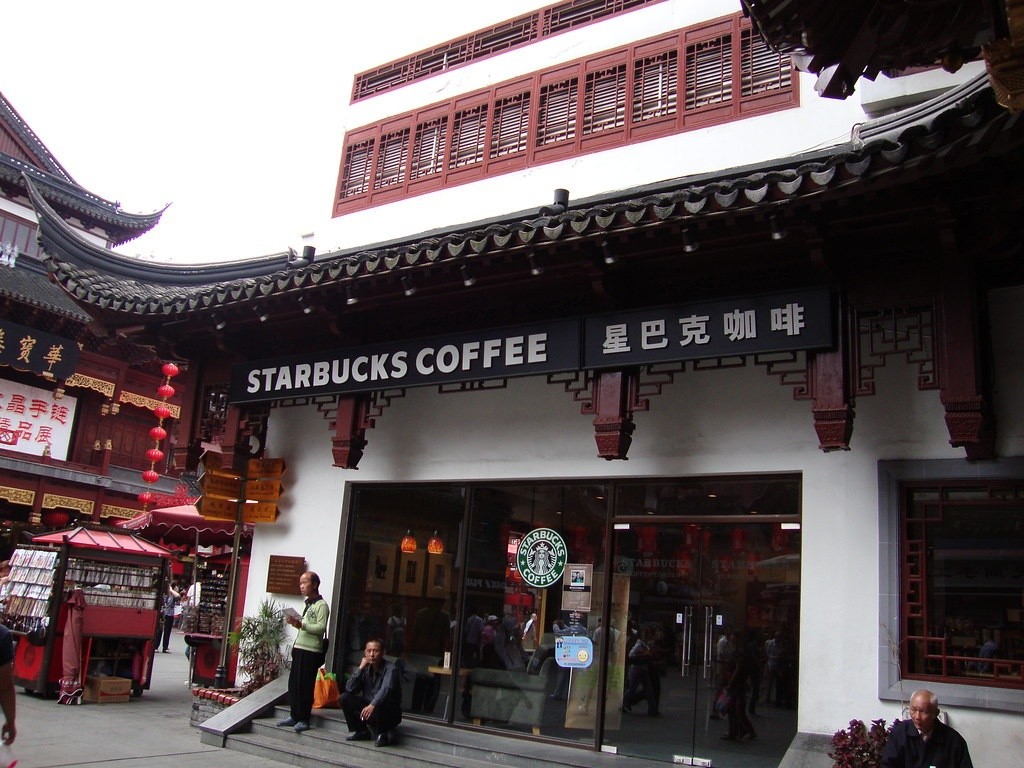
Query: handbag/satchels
xmin=312 ymin=668 xmax=342 ymax=709
xmin=319 ymin=639 xmax=329 ymax=668
xmin=715 ymin=689 xmax=733 ymax=714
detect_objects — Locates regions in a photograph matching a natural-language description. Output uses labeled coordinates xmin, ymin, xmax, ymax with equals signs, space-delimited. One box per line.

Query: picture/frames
xmin=427 ymin=553 xmax=454 ymax=598
xmin=398 ymin=546 xmax=427 ymax=597
xmin=365 ymin=541 xmax=397 ymax=594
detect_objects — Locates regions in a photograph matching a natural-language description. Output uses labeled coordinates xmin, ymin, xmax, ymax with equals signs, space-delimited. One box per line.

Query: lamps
xmin=253 ymin=305 xmax=269 ymax=321
xmin=428 ymin=528 xmax=443 ymax=554
xmin=211 ymin=311 xmax=226 ymax=329
xmin=526 ymin=251 xmax=545 ymax=275
xmin=461 ymin=262 xmax=476 ymax=286
xmin=601 ymin=237 xmax=621 ymax=264
xmin=303 ymin=245 xmax=316 ymax=261
xmin=681 ymin=220 xmax=700 ymax=252
xmin=400 ymin=528 xmax=417 ymax=554
xmin=344 ymin=285 xmax=360 ymax=305
xmin=768 ymin=209 xmax=787 ymax=240
xmin=296 ymin=294 xmax=314 ymax=314
xmin=401 ymin=274 xmax=416 ymax=297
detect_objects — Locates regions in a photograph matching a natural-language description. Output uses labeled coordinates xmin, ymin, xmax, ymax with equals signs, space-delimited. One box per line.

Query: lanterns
xmin=44 ymin=509 xmax=68 ymax=532
xmin=136 ymin=363 xmax=180 ymax=508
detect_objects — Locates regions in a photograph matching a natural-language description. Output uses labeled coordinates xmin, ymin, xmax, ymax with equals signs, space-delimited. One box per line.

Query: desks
xmin=426 ymin=665 xmax=472 ymax=719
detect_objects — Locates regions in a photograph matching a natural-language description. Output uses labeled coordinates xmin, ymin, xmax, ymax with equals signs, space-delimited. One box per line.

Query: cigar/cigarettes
xmin=362 ymin=717 xmax=364 ymax=721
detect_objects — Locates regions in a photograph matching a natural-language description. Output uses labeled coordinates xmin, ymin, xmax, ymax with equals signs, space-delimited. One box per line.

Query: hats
xmin=486 ymin=615 xmax=497 ymax=625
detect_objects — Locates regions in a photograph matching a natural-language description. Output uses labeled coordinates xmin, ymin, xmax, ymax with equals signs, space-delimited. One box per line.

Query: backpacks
xmin=391 ymin=616 xmax=405 ymax=642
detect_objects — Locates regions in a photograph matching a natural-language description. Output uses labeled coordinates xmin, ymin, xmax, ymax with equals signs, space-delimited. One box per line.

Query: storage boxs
xmin=84 ymin=673 xmax=133 ymax=702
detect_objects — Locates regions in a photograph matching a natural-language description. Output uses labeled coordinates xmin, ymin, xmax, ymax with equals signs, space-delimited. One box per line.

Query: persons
xmin=336 ymin=637 xmax=403 ymax=747
xmin=0 ymin=559 xmax=13 ymax=600
xmin=155 ymin=584 xmax=182 ymax=655
xmin=389 ymin=586 xmax=798 ymax=743
xmin=0 ymin=624 xmax=18 ymax=745
xmin=872 ymin=689 xmax=973 ymax=768
xmin=276 ymin=573 xmax=330 ymax=731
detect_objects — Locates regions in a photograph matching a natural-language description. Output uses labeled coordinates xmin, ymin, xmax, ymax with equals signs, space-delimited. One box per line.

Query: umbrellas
xmin=117 ymin=504 xmax=256 ymax=688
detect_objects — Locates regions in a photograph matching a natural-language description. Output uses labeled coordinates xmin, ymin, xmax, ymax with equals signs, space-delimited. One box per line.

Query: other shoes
xmin=749 ymin=711 xmax=762 ymax=718
xmin=710 ymin=714 xmax=721 ymax=719
xmin=163 ymin=650 xmax=170 ymax=653
xmin=346 ymin=731 xmax=372 ymax=741
xmin=277 ymin=716 xmax=297 ymax=726
xmin=746 ymin=731 xmax=758 ymax=740
xmin=375 ymin=733 xmax=389 ymax=747
xmin=720 ymin=735 xmax=737 ymax=741
xmin=155 ymin=649 xmax=159 ymax=653
xmin=648 ymin=711 xmax=662 ymax=716
xmin=294 ymin=721 xmax=310 ymax=731
xmin=623 ymin=703 xmax=633 ymax=714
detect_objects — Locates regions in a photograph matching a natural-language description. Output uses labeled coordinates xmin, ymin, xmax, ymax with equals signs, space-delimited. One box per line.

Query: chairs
xmin=337 ymin=607 xmax=441 ymax=715
xmin=553 ymin=189 xmax=569 ymax=209
xmin=472 ymin=645 xmax=562 ymax=735
